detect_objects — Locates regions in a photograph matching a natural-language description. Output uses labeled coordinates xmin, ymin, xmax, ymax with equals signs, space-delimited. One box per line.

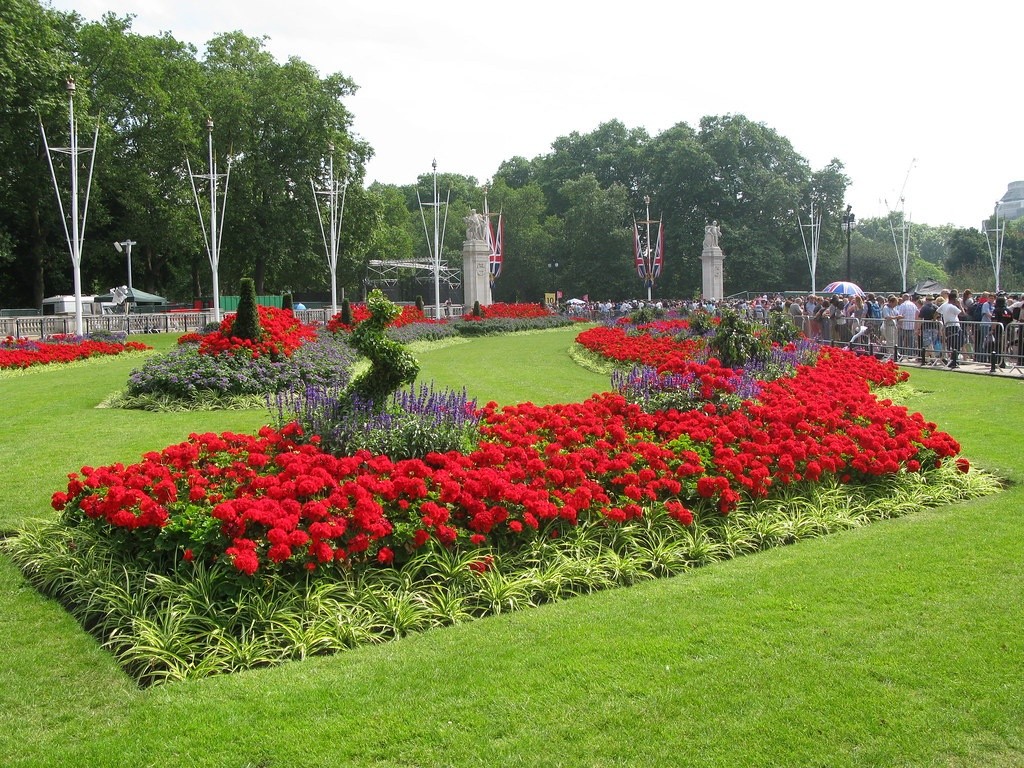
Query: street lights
xmin=992 ymin=200 xmax=1001 ymax=291
xmin=808 ymin=199 xmax=819 ymax=296
xmin=548 ymin=257 xmax=558 ymax=292
xmin=642 ymin=195 xmax=653 ymax=301
xmin=898 ymin=196 xmax=909 ymax=292
xmin=326 ymin=141 xmax=340 ymax=316
xmin=483 ymin=185 xmax=491 ymax=248
xmin=204 ymin=112 xmax=222 ymax=324
xmin=432 ymin=157 xmax=442 ymax=319
xmin=842 ymin=205 xmax=856 ymax=282
xmin=65 ymin=74 xmax=90 ymax=337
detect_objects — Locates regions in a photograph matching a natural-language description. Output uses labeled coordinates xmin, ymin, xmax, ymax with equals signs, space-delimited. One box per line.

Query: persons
xmin=690 ymin=297 xmax=754 ymax=318
xmin=574 ymin=299 xmax=692 ymax=317
xmin=753 ymin=293 xmax=868 ymax=350
xmin=709 ymin=221 xmax=720 ymax=248
xmin=861 ymin=289 xmax=1024 ymax=367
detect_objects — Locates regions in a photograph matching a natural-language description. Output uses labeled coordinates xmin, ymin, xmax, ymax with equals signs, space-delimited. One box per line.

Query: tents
xmin=94 ymin=287 xmax=166 ymax=315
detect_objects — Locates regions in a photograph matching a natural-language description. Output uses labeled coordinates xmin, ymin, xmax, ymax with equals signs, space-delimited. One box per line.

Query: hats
xmin=926 ymin=293 xmax=935 ymax=299
xmin=996 ymin=290 xmax=1009 ymax=295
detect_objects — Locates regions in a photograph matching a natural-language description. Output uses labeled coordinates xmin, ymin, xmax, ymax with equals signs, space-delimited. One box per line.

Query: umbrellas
xmin=821 ymin=282 xmax=868 ymax=297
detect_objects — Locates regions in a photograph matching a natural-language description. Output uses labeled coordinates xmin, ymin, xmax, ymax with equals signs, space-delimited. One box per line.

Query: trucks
xmin=39 ymin=292 xmax=119 ymax=334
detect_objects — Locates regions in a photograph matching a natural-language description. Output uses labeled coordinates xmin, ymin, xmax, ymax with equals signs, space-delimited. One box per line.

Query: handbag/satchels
xmin=982 ymin=333 xmax=995 ymax=350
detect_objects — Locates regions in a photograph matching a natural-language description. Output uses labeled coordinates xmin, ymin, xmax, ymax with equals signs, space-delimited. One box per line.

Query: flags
xmin=632 ymin=212 xmax=663 ymax=288
xmin=489 ymin=211 xmax=504 ymax=288
